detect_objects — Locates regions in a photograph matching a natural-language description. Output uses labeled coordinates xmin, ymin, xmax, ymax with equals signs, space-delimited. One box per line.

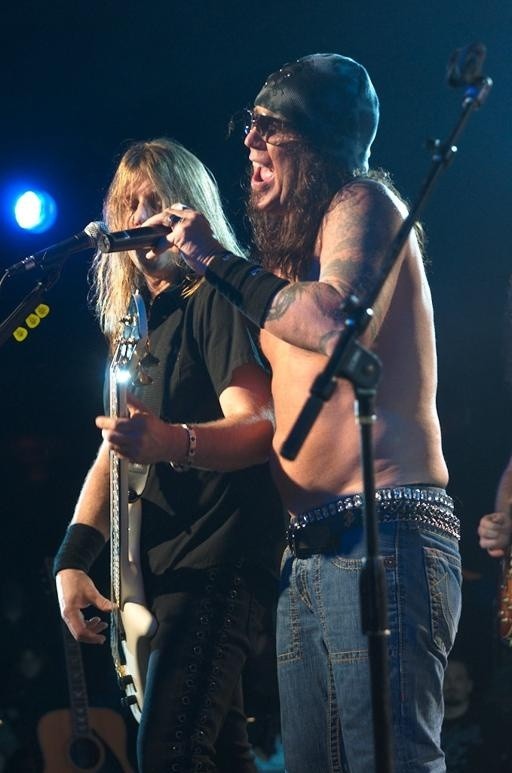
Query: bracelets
xmin=56 ymin=525 xmax=108 ymax=575
xmin=197 ymin=253 xmax=290 ymax=329
xmin=171 ymin=423 xmax=199 ymax=475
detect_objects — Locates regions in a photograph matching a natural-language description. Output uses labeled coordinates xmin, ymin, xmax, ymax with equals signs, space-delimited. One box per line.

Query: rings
xmin=170 ymin=202 xmax=187 ymax=214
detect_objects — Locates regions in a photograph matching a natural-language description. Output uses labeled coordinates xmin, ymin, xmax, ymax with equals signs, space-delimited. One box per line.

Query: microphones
xmin=97 ymin=221 xmax=168 ymax=254
xmin=0 ymin=218 xmax=108 ymax=279
xmin=444 ymin=33 xmax=492 ymax=87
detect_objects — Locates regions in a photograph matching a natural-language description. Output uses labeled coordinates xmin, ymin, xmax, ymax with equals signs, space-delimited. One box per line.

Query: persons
xmin=43 ymin=135 xmax=278 ymax=771
xmin=476 ymin=456 xmax=511 ymax=664
xmin=140 ymin=51 xmax=467 ymax=772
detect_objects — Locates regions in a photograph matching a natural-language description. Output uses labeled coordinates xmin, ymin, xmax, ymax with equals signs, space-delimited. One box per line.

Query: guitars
xmin=37 ymin=555 xmax=132 ymax=772
xmin=110 ymin=295 xmax=159 ymax=723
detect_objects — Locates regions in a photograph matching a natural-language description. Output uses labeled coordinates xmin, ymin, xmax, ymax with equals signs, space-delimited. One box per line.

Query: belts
xmin=284 ymin=505 xmax=463 ymax=557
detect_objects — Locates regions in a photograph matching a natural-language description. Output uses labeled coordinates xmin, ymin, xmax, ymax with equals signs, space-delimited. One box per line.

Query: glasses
xmin=228 ymin=107 xmax=287 ymax=148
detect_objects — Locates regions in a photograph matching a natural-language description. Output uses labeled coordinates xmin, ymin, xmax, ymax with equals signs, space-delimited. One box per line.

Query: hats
xmin=256 ymin=53 xmax=380 ymax=174
